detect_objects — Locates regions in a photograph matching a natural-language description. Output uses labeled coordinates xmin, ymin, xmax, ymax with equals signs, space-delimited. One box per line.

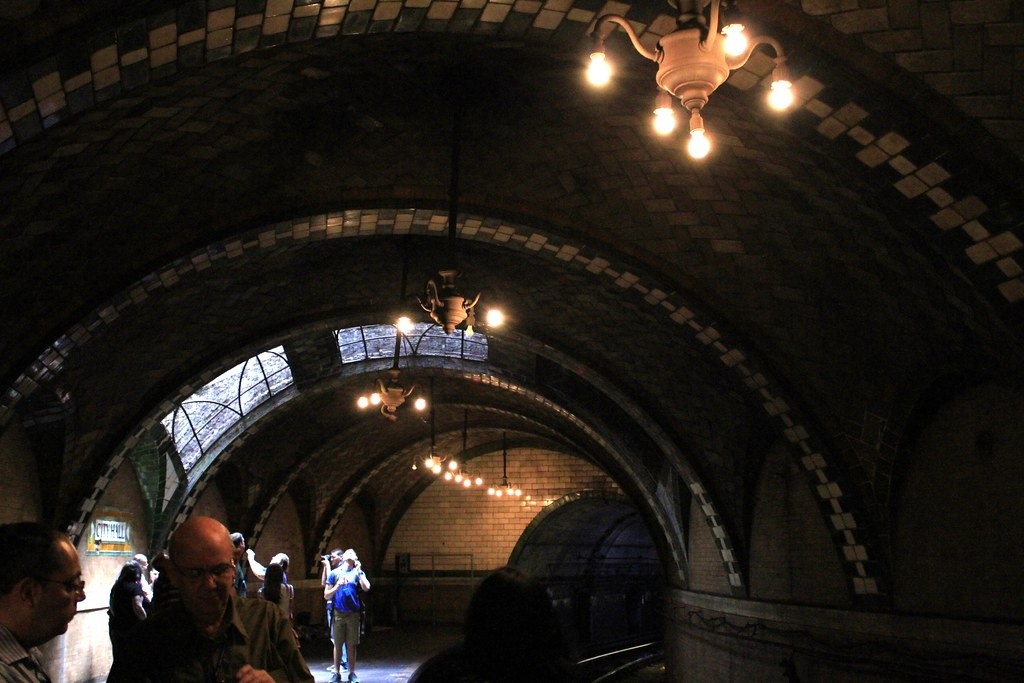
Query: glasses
xmin=174 ymin=557 xmax=236 ymax=582
xmin=31 ymin=574 xmax=85 ymax=593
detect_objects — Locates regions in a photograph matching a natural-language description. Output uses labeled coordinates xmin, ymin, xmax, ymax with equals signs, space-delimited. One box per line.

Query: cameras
xmin=319 ymin=554 xmax=332 ymax=564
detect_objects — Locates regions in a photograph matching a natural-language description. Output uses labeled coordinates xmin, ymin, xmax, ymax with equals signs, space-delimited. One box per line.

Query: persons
xmin=0 ymin=518 xmax=87 ymax=682
xmin=102 ymin=515 xmax=373 ymax=683
xmin=406 ymin=565 xmax=582 ymax=683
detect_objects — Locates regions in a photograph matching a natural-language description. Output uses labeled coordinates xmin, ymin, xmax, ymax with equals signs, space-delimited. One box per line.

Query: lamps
xmin=394 ymin=133 xmax=507 ymax=340
xmin=407 ymin=375 xmax=523 ymax=500
xmin=579 ymin=0 xmax=799 ymax=160
xmin=350 ymin=249 xmax=424 ymax=419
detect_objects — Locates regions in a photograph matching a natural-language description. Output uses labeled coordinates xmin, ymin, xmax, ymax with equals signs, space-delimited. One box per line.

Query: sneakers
xmin=328 ymin=673 xmax=341 ymax=683
xmin=348 ymin=672 xmax=360 ymax=683
